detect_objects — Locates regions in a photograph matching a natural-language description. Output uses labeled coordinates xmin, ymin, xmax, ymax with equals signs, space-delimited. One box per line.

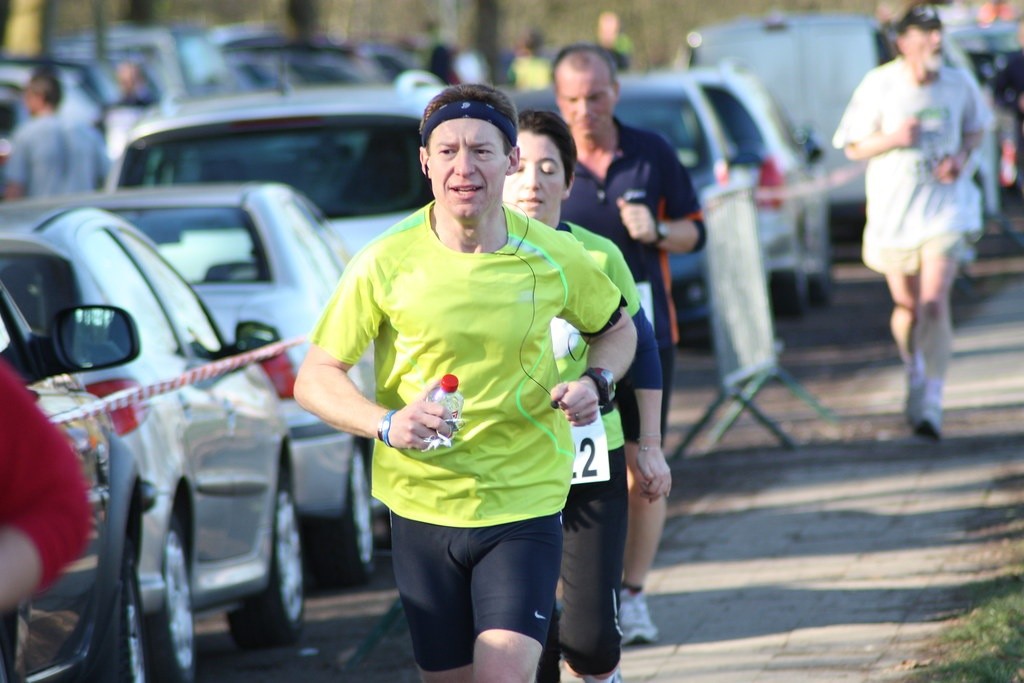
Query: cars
xmin=0 ymin=274 xmax=147 ymax=683
xmin=0 ymin=201 xmax=308 ymax=683
xmin=1 ymin=56 xmax=99 ymax=163
xmin=49 ymin=24 xmax=374 ymax=113
xmin=648 ymin=60 xmax=831 ymax=310
xmin=103 ymin=69 xmax=457 ymax=269
xmin=27 ymin=182 xmax=381 ymax=586
xmin=512 ymin=76 xmax=744 ymax=334
xmin=670 ymin=15 xmax=892 ymax=203
xmin=952 ymin=26 xmax=1024 ymax=109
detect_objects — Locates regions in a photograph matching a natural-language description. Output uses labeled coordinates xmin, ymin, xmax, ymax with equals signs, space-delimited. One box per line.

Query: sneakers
xmin=913 ymin=402 xmax=942 ymax=442
xmin=904 ymin=379 xmax=924 ymax=423
xmin=618 ymin=588 xmax=660 ymax=645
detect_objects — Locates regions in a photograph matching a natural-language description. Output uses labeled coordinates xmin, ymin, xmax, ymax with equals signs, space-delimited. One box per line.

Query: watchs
xmin=579 ymin=367 xmax=616 ymax=405
xmin=647 ymin=222 xmax=669 ymax=247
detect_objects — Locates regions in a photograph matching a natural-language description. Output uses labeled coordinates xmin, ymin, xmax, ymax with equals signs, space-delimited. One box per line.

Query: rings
xmin=573 ymin=412 xmax=579 ymax=423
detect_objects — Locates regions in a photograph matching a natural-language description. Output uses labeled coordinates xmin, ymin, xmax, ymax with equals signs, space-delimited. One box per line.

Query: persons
xmin=0 ymin=359 xmax=99 ymax=683
xmin=835 ymin=2 xmax=991 ymax=441
xmin=595 ymin=11 xmax=641 ymax=79
xmin=552 ymin=48 xmax=704 ymax=644
xmin=3 ymin=75 xmax=110 ymax=203
xmin=453 ymin=38 xmax=491 ymax=86
xmin=296 ymin=84 xmax=639 ymax=683
xmin=427 ymin=28 xmax=458 ymax=86
xmin=502 ymin=108 xmax=672 ymax=683
xmin=507 ymin=29 xmax=553 ymax=90
xmin=95 ymin=60 xmax=157 ymax=135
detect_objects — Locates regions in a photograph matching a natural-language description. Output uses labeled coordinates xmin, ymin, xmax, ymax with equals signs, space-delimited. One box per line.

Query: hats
xmin=893 ymin=4 xmax=943 ymax=32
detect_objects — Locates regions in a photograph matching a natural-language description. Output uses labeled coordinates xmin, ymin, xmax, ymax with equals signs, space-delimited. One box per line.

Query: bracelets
xmin=640 ymin=446 xmax=661 ymax=450
xmin=640 ymin=431 xmax=661 ymax=438
xmin=377 ymin=409 xmax=397 ymax=448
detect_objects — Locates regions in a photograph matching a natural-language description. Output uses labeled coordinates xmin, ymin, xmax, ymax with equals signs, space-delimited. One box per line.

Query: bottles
xmin=425 ymin=374 xmax=464 ymax=445
